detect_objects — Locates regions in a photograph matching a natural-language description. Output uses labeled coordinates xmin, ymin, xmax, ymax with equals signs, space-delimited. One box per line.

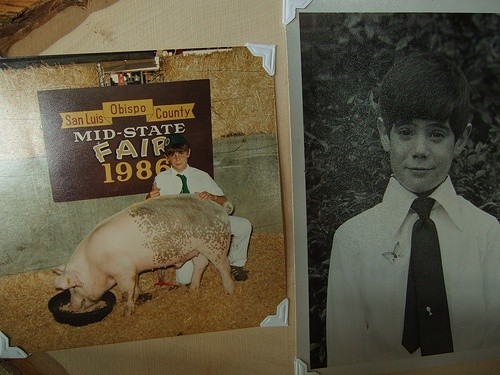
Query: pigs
xmin=52 ymin=195 xmax=236 ymax=318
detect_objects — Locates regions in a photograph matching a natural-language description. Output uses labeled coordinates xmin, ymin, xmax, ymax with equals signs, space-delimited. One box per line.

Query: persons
xmin=145 ymin=133 xmax=253 ymax=287
xmin=327 ymin=50 xmax=500 ymax=369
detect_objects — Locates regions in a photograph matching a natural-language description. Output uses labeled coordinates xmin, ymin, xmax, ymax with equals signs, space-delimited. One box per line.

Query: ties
xmin=176 ymin=173 xmax=190 ymax=194
xmin=400 ymin=198 xmax=454 ymax=356
